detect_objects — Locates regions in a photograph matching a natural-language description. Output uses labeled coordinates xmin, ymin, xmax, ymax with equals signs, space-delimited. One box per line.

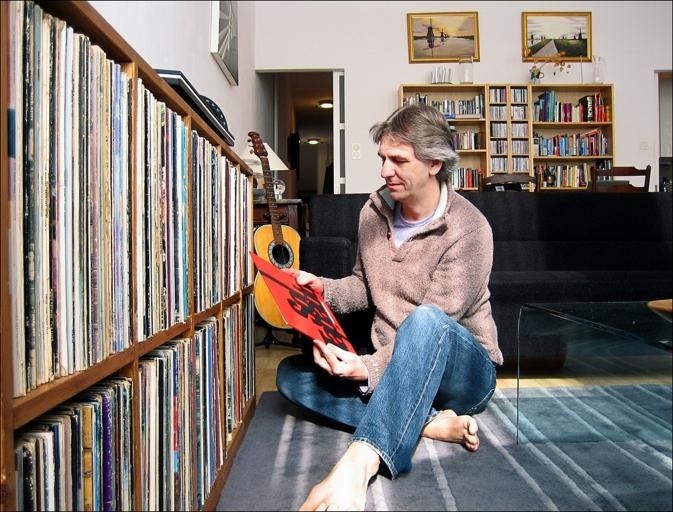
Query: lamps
xmin=240 ymin=140 xmax=290 ymax=197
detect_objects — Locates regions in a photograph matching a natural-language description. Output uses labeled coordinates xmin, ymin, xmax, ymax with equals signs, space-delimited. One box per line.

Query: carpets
xmin=216 ymin=382 xmax=673 ymax=511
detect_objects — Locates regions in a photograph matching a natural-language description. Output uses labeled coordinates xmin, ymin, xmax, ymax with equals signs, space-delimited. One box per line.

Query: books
xmin=447 ymin=121 xmax=488 ymax=152
xmin=515 ymin=141 xmax=531 ymax=159
xmin=241 ymin=160 xmax=254 ymax=291
xmin=514 ymin=157 xmax=538 ymax=175
xmin=532 ymin=126 xmax=614 ymax=155
xmin=399 ymin=84 xmax=487 ymax=120
xmin=139 ymin=331 xmax=193 ymax=511
xmin=493 ymin=157 xmax=509 ymax=175
xmin=492 ymin=124 xmax=511 ymax=142
xmin=133 ymin=75 xmax=190 ymax=344
xmin=450 ymin=158 xmax=488 ymax=190
xmin=13 ymin=367 xmax=137 ymax=512
xmin=250 ymin=251 xmax=360 ymax=363
xmin=242 ymin=291 xmax=254 ymax=414
xmin=223 ymin=153 xmax=238 ymax=304
xmin=224 ymin=298 xmax=241 ymax=454
xmin=511 ymin=86 xmax=529 ymax=104
xmin=197 ymin=312 xmax=224 ymax=510
xmin=532 ymin=154 xmax=616 ymax=191
xmin=491 ymin=107 xmax=509 ymax=121
xmin=189 ymin=120 xmax=219 ymax=317
xmin=513 ymin=123 xmax=529 ymax=138
xmin=512 ymin=105 xmax=531 ymax=121
xmin=488 ymin=88 xmax=508 ymax=104
xmin=493 ymin=141 xmax=511 ymax=159
xmin=535 ymin=90 xmax=618 ymax=122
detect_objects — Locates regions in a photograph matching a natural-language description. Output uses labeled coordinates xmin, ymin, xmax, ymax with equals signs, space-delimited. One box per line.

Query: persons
xmin=274 ymin=104 xmax=505 ymax=509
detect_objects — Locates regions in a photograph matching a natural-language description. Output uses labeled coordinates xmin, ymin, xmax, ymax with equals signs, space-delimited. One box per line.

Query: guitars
xmin=249 ymin=131 xmax=303 ymax=332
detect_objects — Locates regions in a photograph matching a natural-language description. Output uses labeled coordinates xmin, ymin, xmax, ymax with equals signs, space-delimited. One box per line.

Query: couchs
xmin=298 ymin=191 xmax=673 ymax=360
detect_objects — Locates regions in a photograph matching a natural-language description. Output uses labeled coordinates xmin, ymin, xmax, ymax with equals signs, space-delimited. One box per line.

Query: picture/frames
xmin=406 ymin=12 xmax=480 ymax=63
xmin=520 ymin=11 xmax=592 ymax=64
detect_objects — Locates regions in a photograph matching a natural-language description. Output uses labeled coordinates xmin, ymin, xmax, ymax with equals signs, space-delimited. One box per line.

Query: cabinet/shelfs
xmin=396 ymin=83 xmax=615 ymax=189
xmin=0 ymin=1 xmax=259 ymax=511
xmin=253 ymin=197 xmax=302 ymax=233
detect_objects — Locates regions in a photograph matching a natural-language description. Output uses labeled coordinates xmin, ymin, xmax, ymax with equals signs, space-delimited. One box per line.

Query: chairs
xmin=590 ymin=165 xmax=651 ymax=191
